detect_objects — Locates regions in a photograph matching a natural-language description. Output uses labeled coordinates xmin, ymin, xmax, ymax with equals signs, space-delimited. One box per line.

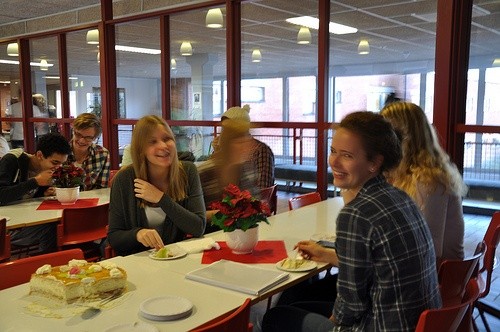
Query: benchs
xmin=274 ymin=163 xmax=340 ymax=196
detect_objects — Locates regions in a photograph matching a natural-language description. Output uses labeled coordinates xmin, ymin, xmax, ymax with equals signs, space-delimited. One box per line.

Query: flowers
xmin=208 ymin=182 xmax=272 ymax=232
xmin=46 ymin=162 xmax=88 ymax=189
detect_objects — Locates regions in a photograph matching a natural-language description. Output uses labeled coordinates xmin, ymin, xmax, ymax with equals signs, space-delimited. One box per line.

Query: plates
xmin=311 ymin=232 xmax=336 ymax=242
xmin=140 ymin=296 xmax=193 ymax=321
xmin=149 ymin=248 xmax=187 ymax=260
xmin=41 ymin=196 xmax=57 ymax=200
xmin=276 ymin=259 xmax=317 ymax=271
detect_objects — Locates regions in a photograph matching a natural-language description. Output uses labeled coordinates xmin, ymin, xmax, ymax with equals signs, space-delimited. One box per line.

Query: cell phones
xmin=316 ymin=240 xmax=335 ymax=248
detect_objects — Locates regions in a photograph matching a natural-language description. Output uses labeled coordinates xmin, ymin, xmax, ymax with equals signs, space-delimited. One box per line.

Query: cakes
xmin=28 ymin=259 xmax=127 ymax=303
xmin=280 ymin=258 xmax=306 ymax=269
xmin=156 ymin=247 xmax=168 ymax=258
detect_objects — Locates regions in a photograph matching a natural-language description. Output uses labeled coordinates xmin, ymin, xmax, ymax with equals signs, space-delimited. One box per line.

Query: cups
xmin=49 ymin=179 xmax=56 ymax=195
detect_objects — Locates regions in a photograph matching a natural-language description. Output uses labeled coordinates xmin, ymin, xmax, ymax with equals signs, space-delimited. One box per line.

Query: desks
xmin=0 ymin=256 xmax=253 ymax=332
xmin=124 ymin=196 xmax=344 ymax=310
xmin=0 ymin=188 xmax=112 ymax=230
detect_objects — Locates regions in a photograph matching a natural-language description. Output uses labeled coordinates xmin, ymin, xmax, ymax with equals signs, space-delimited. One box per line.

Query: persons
xmin=203 ymin=106 xmax=274 ymax=232
xmin=107 ymin=115 xmax=207 ymax=256
xmin=5 ymin=89 xmax=51 ymax=149
xmin=261 ymin=111 xmax=442 ymax=332
xmin=342 ymin=102 xmax=466 ymax=273
xmin=0 ymin=133 xmax=71 ymax=256
xmin=0 ymin=131 xmax=9 ymax=159
xmin=51 ymin=113 xmax=110 ymax=191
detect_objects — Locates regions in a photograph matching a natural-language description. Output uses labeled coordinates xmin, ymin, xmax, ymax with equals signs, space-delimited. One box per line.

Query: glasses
xmin=73 ymin=129 xmax=98 ymax=142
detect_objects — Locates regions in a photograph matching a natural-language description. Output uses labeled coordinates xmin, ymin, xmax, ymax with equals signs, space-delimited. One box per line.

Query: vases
xmin=223 ymin=224 xmax=259 ymax=255
xmin=55 ymin=186 xmax=81 ymax=205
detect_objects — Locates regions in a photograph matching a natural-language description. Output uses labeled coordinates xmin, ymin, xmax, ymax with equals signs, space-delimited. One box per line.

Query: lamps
xmin=179 ymin=41 xmax=192 ymax=55
xmin=297 ymin=27 xmax=312 ymax=44
xmin=356 ymin=39 xmax=370 ymax=55
xmin=40 ymin=57 xmax=49 ymax=71
xmin=86 ymin=29 xmax=98 ymax=44
xmin=7 ymin=42 xmax=19 ymax=57
xmin=252 ymin=48 xmax=261 ymax=62
xmin=205 ymin=8 xmax=223 ymax=29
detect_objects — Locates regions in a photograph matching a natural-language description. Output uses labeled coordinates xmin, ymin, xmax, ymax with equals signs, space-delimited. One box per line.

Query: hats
xmin=222 ymin=104 xmax=251 ymax=130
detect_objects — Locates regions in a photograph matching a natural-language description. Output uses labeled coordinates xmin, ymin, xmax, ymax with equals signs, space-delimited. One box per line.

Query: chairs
xmin=254 ymin=185 xmax=278 ymax=216
xmin=188 ymin=298 xmax=254 ymax=332
xmin=0 ymin=202 xmax=116 ymax=292
xmin=415 ymin=209 xmax=500 ymax=332
xmin=287 ymin=191 xmax=321 ymax=211
